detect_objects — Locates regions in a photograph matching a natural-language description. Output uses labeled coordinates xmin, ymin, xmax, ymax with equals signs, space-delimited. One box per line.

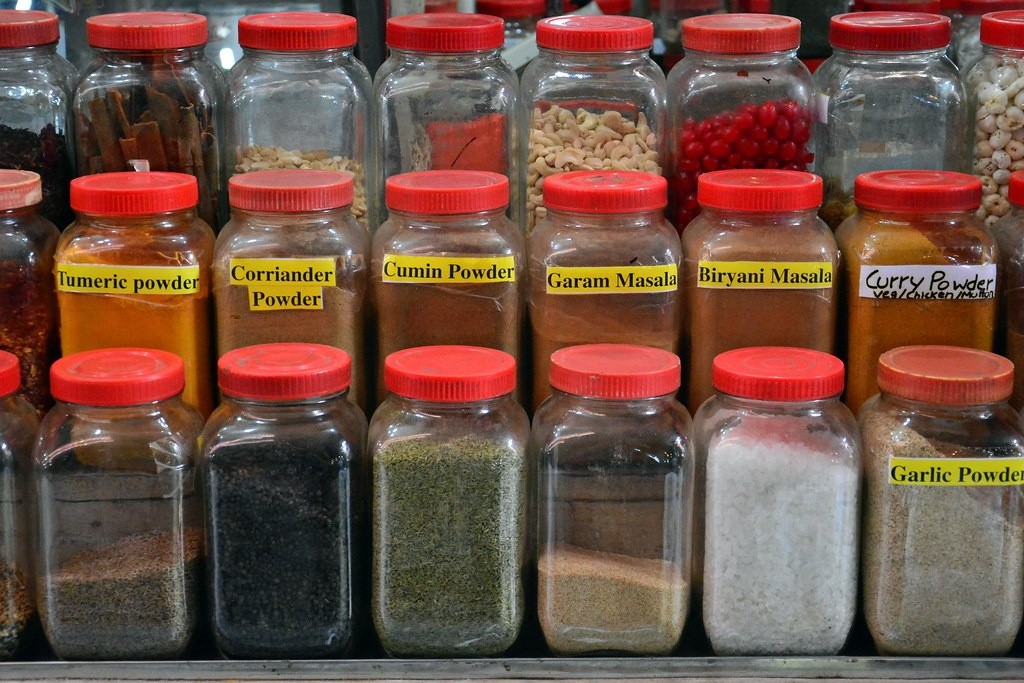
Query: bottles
xmin=691 ymin=345 xmax=863 ymax=655
xmin=367 ymin=344 xmax=530 ymax=658
xmin=29 ymin=346 xmax=205 ymax=661
xmin=211 ymin=167 xmax=371 ymax=412
xmin=680 ymin=168 xmax=840 ymax=416
xmin=53 ymin=169 xmax=216 ymax=473
xmin=522 ymin=164 xmax=684 ymax=419
xmin=69 ymin=12 xmax=228 ymax=237
xmin=530 ymin=342 xmax=696 ymax=655
xmin=0 ymin=8 xmax=81 ymax=656
xmin=375 ymin=0 xmax=1024 ymax=239
xmin=201 ymin=341 xmax=367 ymax=660
xmin=834 ymin=170 xmax=1001 ymax=419
xmin=989 ymin=168 xmax=1024 ymax=414
xmin=856 ymin=345 xmax=1024 ymax=658
xmin=225 ymin=5 xmax=374 ymax=237
xmin=369 ymin=171 xmax=527 ymax=410
